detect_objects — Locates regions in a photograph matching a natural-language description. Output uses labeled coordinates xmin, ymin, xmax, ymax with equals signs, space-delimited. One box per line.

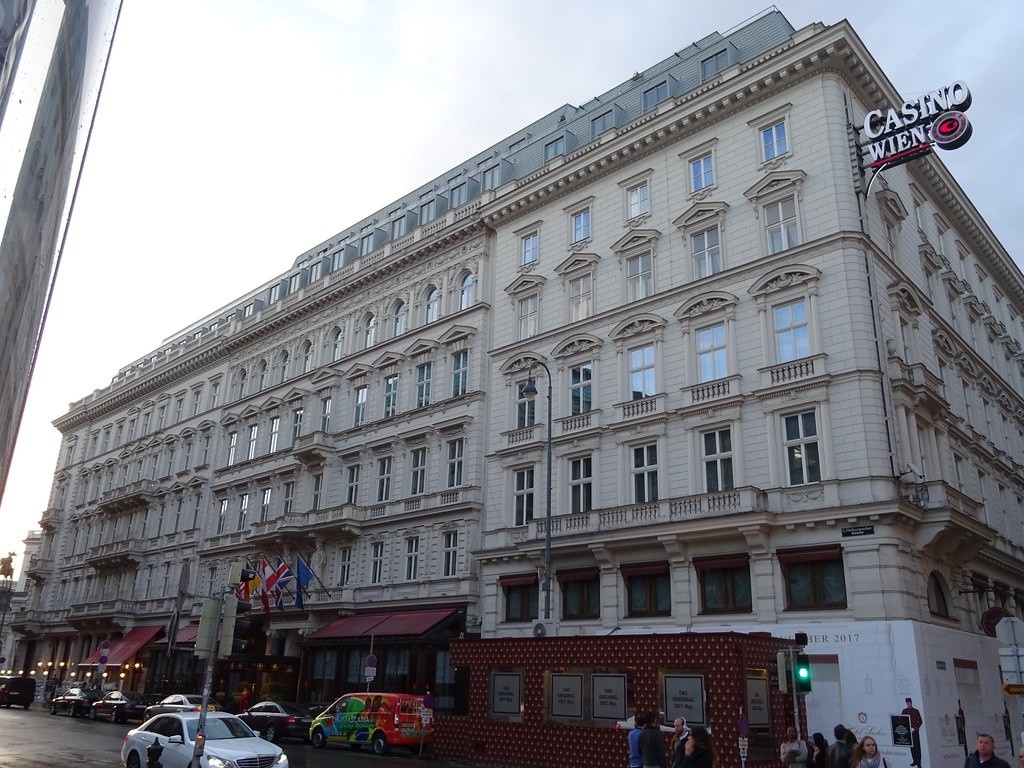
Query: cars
xmin=120 ymin=712 xmax=289 ymax=768
xmin=233 ymin=700 xmax=314 ymax=742
xmin=49 ymin=688 xmax=105 ymax=718
xmin=143 ymin=694 xmax=225 ymax=723
xmin=89 ymin=690 xmax=162 ymax=723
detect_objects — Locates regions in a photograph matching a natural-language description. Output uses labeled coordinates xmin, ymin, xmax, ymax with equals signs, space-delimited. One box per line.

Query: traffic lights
xmin=796 ymin=655 xmax=811 ymax=692
xmin=795 ymin=633 xmax=807 ymax=645
xmin=217 ymin=595 xmax=252 ymax=661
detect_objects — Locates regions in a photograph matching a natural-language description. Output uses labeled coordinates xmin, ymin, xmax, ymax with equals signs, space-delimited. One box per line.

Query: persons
xmin=780 ymin=725 xmax=885 ymax=768
xmin=50 ymin=674 xmax=58 ymax=700
xmin=239 ymin=686 xmax=250 ymax=713
xmin=964 ymin=734 xmax=1010 ymax=768
xmin=629 ymin=712 xmax=719 ymax=768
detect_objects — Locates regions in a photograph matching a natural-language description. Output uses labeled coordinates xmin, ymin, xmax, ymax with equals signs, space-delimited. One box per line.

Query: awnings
xmin=78 ymin=625 xmax=165 ymax=666
xmin=309 ymin=608 xmax=459 ymax=642
xmin=157 ymin=624 xmax=223 ymax=643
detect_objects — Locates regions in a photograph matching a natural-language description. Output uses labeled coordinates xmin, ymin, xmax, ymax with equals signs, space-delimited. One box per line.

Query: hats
xmin=905 ymin=698 xmax=912 ymax=703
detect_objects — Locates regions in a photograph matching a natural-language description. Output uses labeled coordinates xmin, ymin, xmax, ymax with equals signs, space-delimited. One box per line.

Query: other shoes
xmin=910 ymin=761 xmax=917 ymax=766
xmin=917 ymin=764 xmax=922 ymax=768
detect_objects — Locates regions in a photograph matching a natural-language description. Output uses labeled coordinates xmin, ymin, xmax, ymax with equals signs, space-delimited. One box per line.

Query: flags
xmin=296 ymin=552 xmax=314 ymax=611
xmin=259 ymin=557 xmax=277 ymax=618
xmin=275 ymin=555 xmax=294 ymax=611
xmin=236 ymin=560 xmax=262 ymax=602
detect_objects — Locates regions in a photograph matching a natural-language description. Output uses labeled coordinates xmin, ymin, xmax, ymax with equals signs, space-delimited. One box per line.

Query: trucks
xmin=434 ymin=631 xmax=806 ymax=768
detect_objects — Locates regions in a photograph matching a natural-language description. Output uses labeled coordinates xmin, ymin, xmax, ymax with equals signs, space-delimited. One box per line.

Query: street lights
xmin=521 ymin=359 xmax=552 ymax=618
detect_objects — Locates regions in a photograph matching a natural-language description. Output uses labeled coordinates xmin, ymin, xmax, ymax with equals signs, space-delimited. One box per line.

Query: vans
xmin=309 ymin=692 xmax=434 ymax=755
xmin=0 ymin=676 xmax=36 ymax=709
